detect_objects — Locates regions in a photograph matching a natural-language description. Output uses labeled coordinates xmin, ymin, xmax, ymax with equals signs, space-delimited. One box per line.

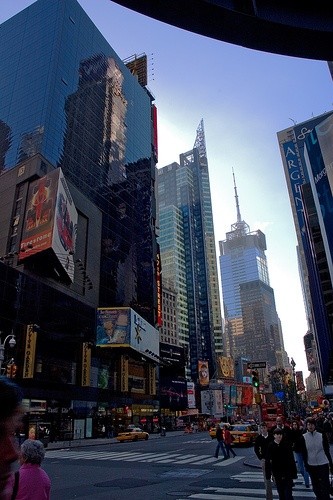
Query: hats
xmin=273 ymin=428 xmax=282 ymax=434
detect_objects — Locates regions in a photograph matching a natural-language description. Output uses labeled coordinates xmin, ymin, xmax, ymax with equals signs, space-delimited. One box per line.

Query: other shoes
xmin=306 ymin=485 xmax=309 ymax=488
xmin=274 ymin=485 xmax=276 ymax=487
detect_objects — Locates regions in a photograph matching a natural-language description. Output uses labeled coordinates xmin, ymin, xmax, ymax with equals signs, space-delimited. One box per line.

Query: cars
xmin=116 ymin=424 xmax=149 ymax=442
xmin=228 ymin=419 xmax=260 ymax=448
xmin=209 ymin=421 xmax=230 ymax=439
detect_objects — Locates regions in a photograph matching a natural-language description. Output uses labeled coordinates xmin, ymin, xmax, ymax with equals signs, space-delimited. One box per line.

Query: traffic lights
xmin=252 ymin=370 xmax=259 ymax=388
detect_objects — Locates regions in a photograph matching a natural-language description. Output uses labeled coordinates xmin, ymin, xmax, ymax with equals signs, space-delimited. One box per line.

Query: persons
xmin=198 ymin=362 xmax=208 ymax=384
xmin=39 ymin=424 xmax=51 ymax=449
xmin=243 ymin=376 xmax=252 ymax=384
xmin=271 ymin=406 xmax=333 ymax=450
xmin=222 ymin=425 xmax=237 ymax=459
xmin=212 ymin=424 xmax=226 ymax=459
xmin=191 ymin=417 xmax=217 ymax=433
xmin=265 ymin=428 xmax=295 ymax=500
xmin=1 ymin=439 xmax=51 ymax=500
xmin=254 ymin=419 xmax=278 ymax=500
xmin=300 ymin=417 xmax=332 ymax=500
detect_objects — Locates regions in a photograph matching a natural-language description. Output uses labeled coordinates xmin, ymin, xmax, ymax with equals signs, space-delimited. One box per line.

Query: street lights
xmin=291 ymin=358 xmax=296 ymax=411
xmin=0 ymin=334 xmax=16 ymax=366
